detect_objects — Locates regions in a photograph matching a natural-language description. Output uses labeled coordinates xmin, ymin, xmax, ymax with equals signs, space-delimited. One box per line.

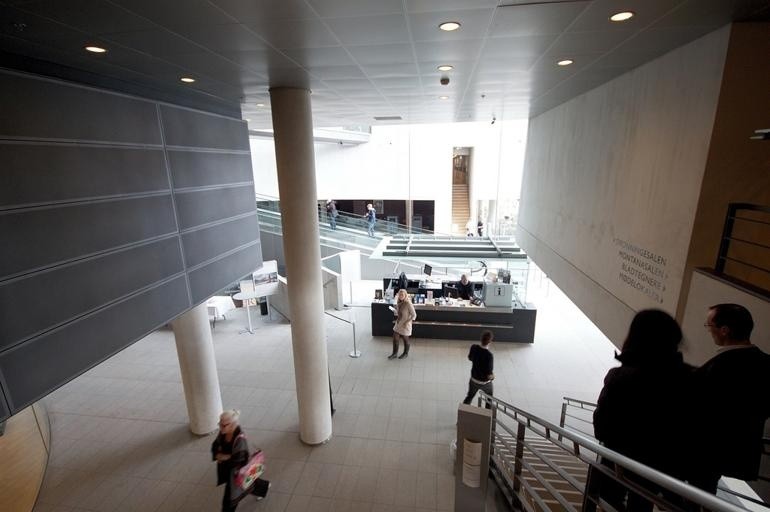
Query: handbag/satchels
xmin=229 ymin=450 xmax=265 ymax=500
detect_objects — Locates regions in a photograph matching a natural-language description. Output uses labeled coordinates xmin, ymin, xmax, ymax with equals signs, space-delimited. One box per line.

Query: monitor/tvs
xmin=443 ymin=287 xmax=458 ymax=304
xmin=424 ymin=264 xmax=432 ymax=276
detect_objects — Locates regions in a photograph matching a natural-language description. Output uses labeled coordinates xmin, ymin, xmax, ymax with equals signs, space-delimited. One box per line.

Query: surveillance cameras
xmin=440 ymin=76 xmax=449 ymax=85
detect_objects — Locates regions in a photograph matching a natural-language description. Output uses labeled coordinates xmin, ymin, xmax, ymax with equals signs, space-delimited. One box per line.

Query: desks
xmin=208 ymin=260 xmax=278 ymax=334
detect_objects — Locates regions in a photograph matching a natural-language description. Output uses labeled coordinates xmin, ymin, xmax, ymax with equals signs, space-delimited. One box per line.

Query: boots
xmin=388 ymin=340 xmax=399 ymax=359
xmin=399 ymin=344 xmax=411 ymax=359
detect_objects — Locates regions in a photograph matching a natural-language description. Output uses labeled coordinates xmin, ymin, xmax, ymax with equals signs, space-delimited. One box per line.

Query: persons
xmin=466 ymin=217 xmax=474 ymax=236
xmin=463 ymin=331 xmax=493 ymax=410
xmin=585 ymin=309 xmax=716 ymax=512
xmin=363 ymin=203 xmax=377 ymax=238
xmin=387 ymin=288 xmax=417 ymax=359
xmin=701 ymin=304 xmax=769 ymax=512
xmin=326 ymin=200 xmax=339 ymax=233
xmin=477 ymin=216 xmax=484 ymax=236
xmin=209 ymin=409 xmax=272 ymax=512
xmin=457 ymin=274 xmax=474 ymax=300
